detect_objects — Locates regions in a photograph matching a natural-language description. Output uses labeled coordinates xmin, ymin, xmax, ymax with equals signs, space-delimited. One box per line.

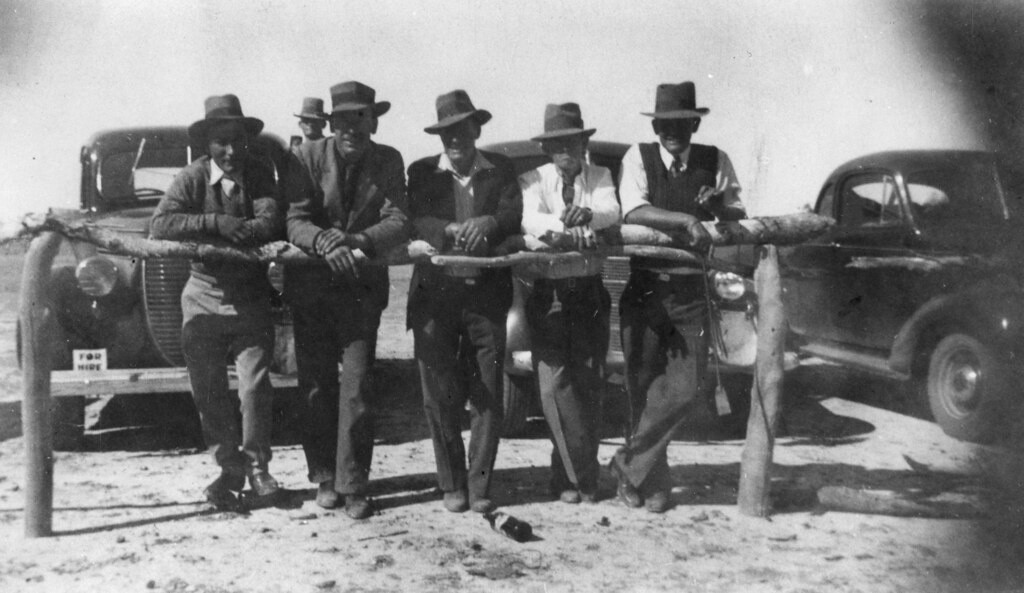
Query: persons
xmin=405 ymin=88 xmax=522 ymax=511
xmin=292 ymin=97 xmax=329 ymax=139
xmin=610 ymin=82 xmax=747 ymax=512
xmin=519 ymin=102 xmax=621 ymax=501
xmin=285 ymin=81 xmax=407 ymax=520
xmin=152 ymin=95 xmax=290 ymax=500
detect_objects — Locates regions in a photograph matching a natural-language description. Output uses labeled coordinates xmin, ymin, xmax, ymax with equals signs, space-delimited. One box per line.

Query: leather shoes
xmin=647 ymin=469 xmax=672 ymax=513
xmin=609 ymin=455 xmax=642 ymax=510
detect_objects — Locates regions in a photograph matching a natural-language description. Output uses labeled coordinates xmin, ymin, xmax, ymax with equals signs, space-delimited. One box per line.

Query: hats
xmin=530 ymin=103 xmax=595 ymax=142
xmin=323 ymin=82 xmax=390 ymax=121
xmin=425 ymin=90 xmax=492 ymax=134
xmin=187 ymin=94 xmax=264 ymax=145
xmin=641 ymin=81 xmax=709 ymax=120
xmin=294 ymin=97 xmax=330 ymax=121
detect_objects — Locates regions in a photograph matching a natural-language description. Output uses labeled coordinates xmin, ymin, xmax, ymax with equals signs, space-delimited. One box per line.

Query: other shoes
xmin=345 ymin=492 xmax=369 ymax=520
xmin=468 ymin=486 xmax=492 ymax=512
xmin=204 ymin=472 xmax=245 ymax=502
xmin=444 ymin=487 xmax=466 ymax=512
xmin=580 ymin=482 xmax=598 ymax=504
xmin=551 ymin=477 xmax=580 ymax=502
xmin=248 ymin=471 xmax=283 ymax=509
xmin=317 ymin=480 xmax=338 ymax=509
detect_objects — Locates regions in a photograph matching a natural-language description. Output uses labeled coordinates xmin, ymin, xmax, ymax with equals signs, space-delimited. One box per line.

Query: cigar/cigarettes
xmin=461 ymin=237 xmax=465 ymax=241
xmin=584 ymin=231 xmax=591 ymax=237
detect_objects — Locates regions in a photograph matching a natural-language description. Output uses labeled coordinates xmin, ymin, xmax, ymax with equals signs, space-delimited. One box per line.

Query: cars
xmin=461 ymin=140 xmax=801 ymax=439
xmin=778 ymin=148 xmax=1024 ymax=444
xmin=16 ymin=127 xmax=303 ymax=448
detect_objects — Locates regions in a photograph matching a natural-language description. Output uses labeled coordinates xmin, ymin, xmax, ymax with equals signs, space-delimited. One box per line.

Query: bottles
xmin=482 ymin=510 xmax=533 ymax=543
